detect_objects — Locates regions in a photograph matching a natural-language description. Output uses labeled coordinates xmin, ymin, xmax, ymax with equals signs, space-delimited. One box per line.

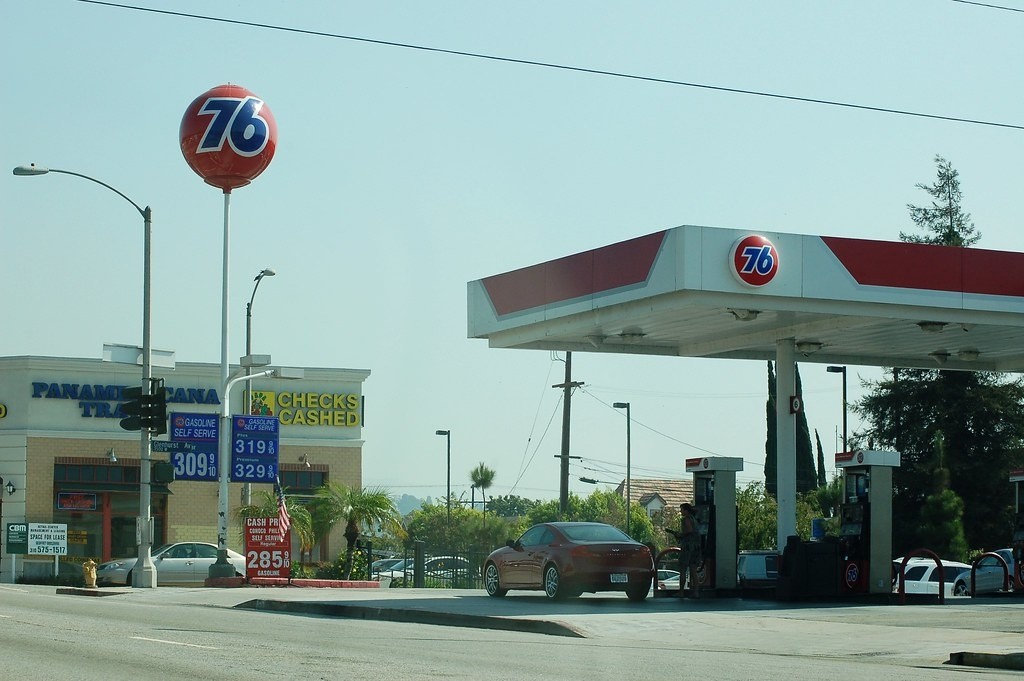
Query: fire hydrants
xmin=82 ymin=557 xmax=98 ymax=589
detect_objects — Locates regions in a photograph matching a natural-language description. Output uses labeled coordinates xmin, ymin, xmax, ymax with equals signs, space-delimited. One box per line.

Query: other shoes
xmin=688 ymin=592 xmax=700 ymax=599
xmin=673 ymin=591 xmax=684 ymax=598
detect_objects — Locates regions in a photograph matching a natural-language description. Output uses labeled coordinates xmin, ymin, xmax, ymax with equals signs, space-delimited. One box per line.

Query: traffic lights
xmin=142 ymin=386 xmax=167 ymax=435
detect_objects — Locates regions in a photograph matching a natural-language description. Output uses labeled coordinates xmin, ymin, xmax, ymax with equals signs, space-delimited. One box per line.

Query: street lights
xmin=827 ymin=366 xmax=846 ymax=452
xmin=242 ymin=267 xmax=277 ymax=554
xmin=13 ymin=163 xmax=157 ymax=589
xmin=435 ymin=430 xmax=451 ymax=523
xmin=208 ymin=362 xmax=305 ymax=578
xmin=613 ymin=403 xmax=631 ymax=535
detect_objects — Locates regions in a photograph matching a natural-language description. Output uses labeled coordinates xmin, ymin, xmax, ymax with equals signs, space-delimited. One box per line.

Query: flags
xmin=277 ymin=478 xmax=290 ymax=542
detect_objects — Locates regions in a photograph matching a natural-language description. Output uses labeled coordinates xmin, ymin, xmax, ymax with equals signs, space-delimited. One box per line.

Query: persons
xmin=671 ymin=503 xmax=701 ymax=597
xmin=178 ymin=550 xmax=187 ymax=557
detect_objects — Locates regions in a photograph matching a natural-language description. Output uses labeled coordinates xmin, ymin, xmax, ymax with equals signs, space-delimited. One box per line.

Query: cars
xmin=96 ymin=541 xmax=247 ymax=587
xmin=737 ymin=550 xmax=780 ymax=586
xmin=483 ymin=521 xmax=655 ymax=602
xmin=950 ymin=547 xmax=1024 ymax=596
xmin=892 ymin=557 xmax=973 ymax=598
xmin=371 ymin=555 xmax=483 ymax=588
xmin=15 ymin=560 xmax=86 ymax=588
xmin=650 ymin=569 xmax=705 ymax=590
